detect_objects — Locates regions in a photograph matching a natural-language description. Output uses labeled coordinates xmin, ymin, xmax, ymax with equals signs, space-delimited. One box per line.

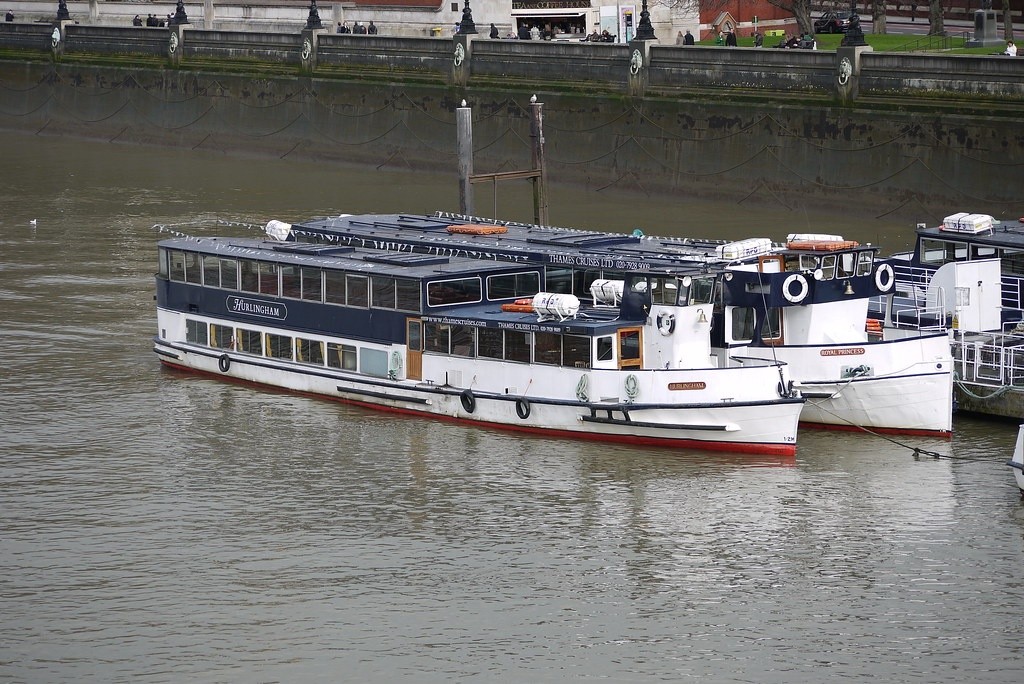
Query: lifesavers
xmin=460 ymin=389 xmax=475 ymax=413
xmin=218 ymin=354 xmax=230 ymax=372
xmin=501 ymin=299 xmax=534 ymax=312
xmin=515 ymin=397 xmax=530 ymax=419
xmin=866 ymin=319 xmax=882 ymax=330
xmin=782 ymin=273 xmax=809 ymax=303
xmin=446 ymin=225 xmax=507 ymax=235
xmin=875 ymin=263 xmax=894 ymax=292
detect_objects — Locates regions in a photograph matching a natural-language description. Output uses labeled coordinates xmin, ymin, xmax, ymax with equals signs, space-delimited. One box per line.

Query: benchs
xmin=34 ymin=16 xmax=57 ymax=23
xmin=579 ymin=34 xmax=617 ymax=42
xmin=771 ymin=41 xmax=814 ymax=49
xmin=998 ymin=49 xmax=1024 ymax=56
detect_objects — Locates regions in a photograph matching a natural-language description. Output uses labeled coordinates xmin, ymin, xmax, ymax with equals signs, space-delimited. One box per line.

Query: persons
xmin=751 ymin=31 xmax=763 ymax=48
xmin=725 ymin=27 xmax=738 ymax=47
xmin=454 ymin=21 xmax=459 ymax=32
xmin=5 ymin=9 xmax=14 ymax=22
xmin=132 ymin=15 xmax=143 ymax=27
xmin=490 ymin=23 xmax=499 ymax=38
xmin=773 ymin=31 xmax=815 ymax=49
xmin=146 ymin=13 xmax=174 ymax=27
xmin=337 ymin=21 xmax=366 ymax=34
xmin=580 ymin=27 xmax=610 ymax=42
xmin=716 ymin=30 xmax=725 ymax=46
xmin=999 ymin=40 xmax=1017 ymax=56
xmin=675 ymin=30 xmax=694 ymax=45
xmin=368 ymin=21 xmax=377 ymax=35
xmin=518 ymin=20 xmax=584 ymax=40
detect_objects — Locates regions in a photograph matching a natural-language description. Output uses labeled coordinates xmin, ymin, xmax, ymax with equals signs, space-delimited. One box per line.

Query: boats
xmin=152 ymin=102 xmax=1024 ymax=494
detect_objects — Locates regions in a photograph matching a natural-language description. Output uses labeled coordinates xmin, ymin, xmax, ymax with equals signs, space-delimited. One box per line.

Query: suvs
xmin=814 ymin=12 xmax=854 ymax=34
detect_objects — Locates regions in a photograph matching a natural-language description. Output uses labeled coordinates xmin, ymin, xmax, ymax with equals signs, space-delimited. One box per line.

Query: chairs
xmin=575 ymin=361 xmax=590 ymax=368
